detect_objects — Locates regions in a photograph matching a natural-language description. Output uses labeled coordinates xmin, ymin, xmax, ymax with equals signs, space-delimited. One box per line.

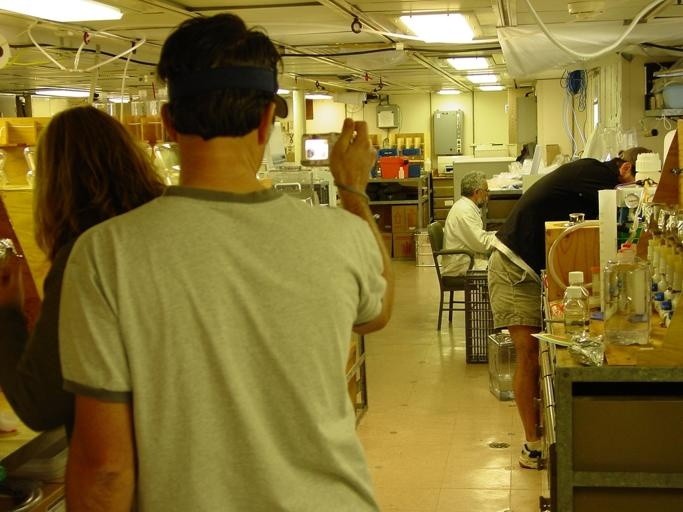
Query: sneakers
xmin=519 ymin=443 xmax=546 ymax=469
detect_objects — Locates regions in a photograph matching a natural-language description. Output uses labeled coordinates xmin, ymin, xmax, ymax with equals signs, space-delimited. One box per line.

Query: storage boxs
xmin=394 ymin=233 xmax=416 ymax=258
xmin=391 ymin=206 xmax=417 ymax=233
xmin=414 ymin=232 xmax=442 ymax=267
xmin=464 ymin=270 xmax=494 ymax=363
xmin=380 ymin=233 xmax=393 ymax=258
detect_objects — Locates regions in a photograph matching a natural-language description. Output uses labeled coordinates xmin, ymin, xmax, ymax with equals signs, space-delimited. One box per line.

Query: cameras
xmin=300 ymin=133 xmax=353 ymax=167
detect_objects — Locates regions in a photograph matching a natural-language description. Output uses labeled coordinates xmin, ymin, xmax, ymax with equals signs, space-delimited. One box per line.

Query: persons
xmin=440 ymin=171 xmax=496 ymax=277
xmin=57 ymin=14 xmax=397 ymax=511
xmin=487 ymin=146 xmax=653 ymax=471
xmin=0 ymin=105 xmax=167 ymax=446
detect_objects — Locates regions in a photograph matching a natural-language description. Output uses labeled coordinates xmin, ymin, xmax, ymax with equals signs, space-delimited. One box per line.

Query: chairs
xmin=427 ymin=222 xmax=474 ymax=331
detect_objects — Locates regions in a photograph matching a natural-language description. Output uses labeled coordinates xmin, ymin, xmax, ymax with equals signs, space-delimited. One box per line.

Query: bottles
xmin=398 ymin=166 xmax=405 ymax=179
xmin=563 ymin=286 xmax=586 ymax=341
xmin=564 ymin=270 xmax=591 ymax=335
xmin=603 ymin=243 xmax=655 ymax=345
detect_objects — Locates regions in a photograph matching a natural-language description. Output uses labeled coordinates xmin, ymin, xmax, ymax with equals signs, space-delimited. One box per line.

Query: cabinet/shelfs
xmin=432 ymin=169 xmax=453 ymax=226
xmin=366 ymin=174 xmax=431 ymax=228
xmin=483 ymin=189 xmax=523 ymax=232
xmin=538 ymin=269 xmax=682 ymax=512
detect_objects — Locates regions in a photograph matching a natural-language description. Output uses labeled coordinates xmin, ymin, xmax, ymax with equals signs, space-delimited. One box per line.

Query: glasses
xmin=478 ymin=188 xmax=489 ymax=192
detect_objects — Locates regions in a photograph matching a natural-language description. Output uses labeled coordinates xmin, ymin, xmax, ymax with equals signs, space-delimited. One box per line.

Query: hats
xmin=167 ymin=64 xmax=289 ymax=118
xmin=618 ymin=149 xmax=636 ymax=179
